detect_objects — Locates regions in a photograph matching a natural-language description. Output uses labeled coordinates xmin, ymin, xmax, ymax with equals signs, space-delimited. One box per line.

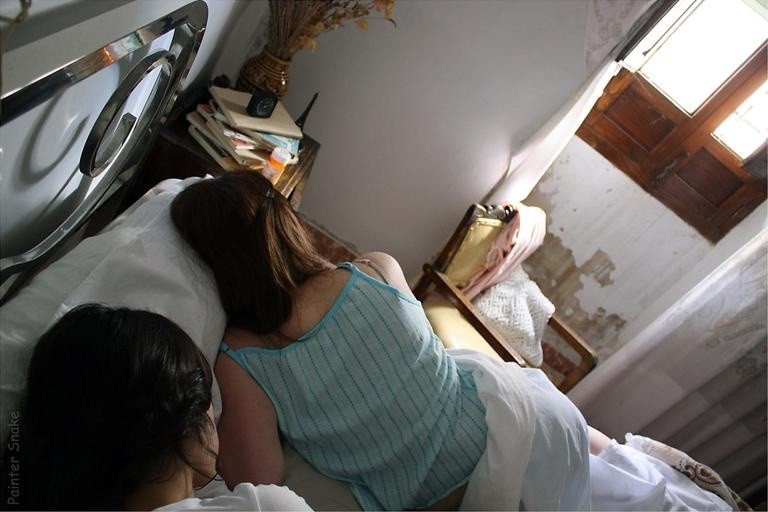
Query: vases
xmin=234 ymin=44 xmax=293 ymax=102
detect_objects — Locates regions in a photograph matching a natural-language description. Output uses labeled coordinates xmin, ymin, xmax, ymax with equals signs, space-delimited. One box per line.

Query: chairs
xmin=412 ymin=202 xmax=599 ymax=396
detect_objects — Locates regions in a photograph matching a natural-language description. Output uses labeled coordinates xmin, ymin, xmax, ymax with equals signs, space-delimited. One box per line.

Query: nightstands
xmin=115 ymin=74 xmax=322 ymax=217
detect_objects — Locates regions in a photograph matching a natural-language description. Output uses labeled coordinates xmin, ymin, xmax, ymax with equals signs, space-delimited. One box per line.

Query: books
xmin=186 ymin=82 xmax=305 ymax=173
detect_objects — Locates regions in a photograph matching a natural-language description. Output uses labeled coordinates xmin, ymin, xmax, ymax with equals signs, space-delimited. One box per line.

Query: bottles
xmin=260 ymin=146 xmax=292 ymax=186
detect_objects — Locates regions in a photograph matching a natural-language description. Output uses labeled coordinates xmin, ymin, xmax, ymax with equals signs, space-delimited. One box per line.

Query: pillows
xmin=0 ymin=172 xmax=231 ymax=459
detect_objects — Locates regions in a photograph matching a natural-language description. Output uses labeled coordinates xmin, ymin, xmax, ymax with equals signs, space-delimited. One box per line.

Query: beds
xmin=0 ymin=0 xmax=754 ymax=512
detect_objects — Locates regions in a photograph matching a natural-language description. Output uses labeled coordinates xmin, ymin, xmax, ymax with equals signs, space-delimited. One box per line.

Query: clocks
xmin=245 ymin=89 xmax=278 ymax=118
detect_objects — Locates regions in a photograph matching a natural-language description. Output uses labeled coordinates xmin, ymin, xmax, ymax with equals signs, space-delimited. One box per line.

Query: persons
xmin=172 ymin=169 xmax=731 ymax=511
xmin=20 ymin=302 xmax=313 ymax=509
